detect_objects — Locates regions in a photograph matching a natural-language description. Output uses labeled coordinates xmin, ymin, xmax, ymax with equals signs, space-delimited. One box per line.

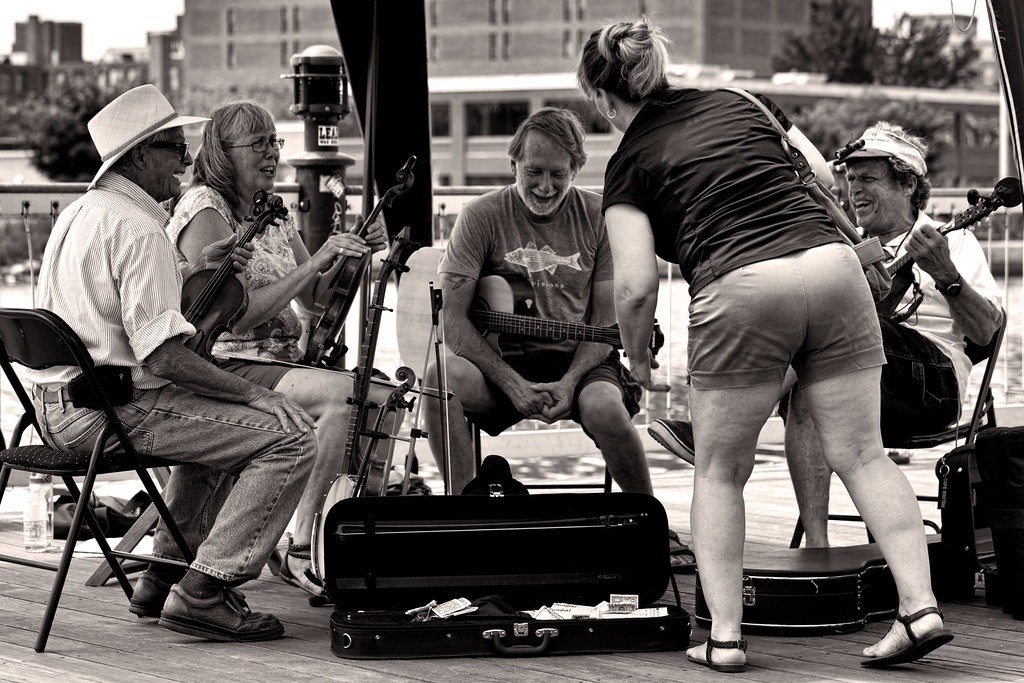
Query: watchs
xmin=934 ymin=272 xmax=963 ymax=297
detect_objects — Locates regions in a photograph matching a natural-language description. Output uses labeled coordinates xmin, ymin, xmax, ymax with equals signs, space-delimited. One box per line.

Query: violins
xmin=176 ymin=188 xmax=289 ymax=366
xmin=300 ymin=154 xmax=418 ymax=370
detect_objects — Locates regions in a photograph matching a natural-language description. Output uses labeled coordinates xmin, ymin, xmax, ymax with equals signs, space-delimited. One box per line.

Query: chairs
xmin=788 ymin=302 xmax=1007 ymax=547
xmin=472 ymin=417 xmax=613 ymax=492
xmin=0 ymin=307 xmax=225 ymax=653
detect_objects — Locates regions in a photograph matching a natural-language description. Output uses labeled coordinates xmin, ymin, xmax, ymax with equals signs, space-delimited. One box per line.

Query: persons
xmin=649 ymin=121 xmax=1003 ymax=548
xmin=27 ymin=84 xmax=319 ymax=642
xmin=418 ymin=106 xmax=697 ymax=571
xmin=163 ymin=101 xmax=407 ymax=597
xmin=576 ymin=20 xmax=955 ymax=671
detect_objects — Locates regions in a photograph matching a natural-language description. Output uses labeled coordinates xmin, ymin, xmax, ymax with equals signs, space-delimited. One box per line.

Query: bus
xmin=338 ymin=63 xmax=757 ymax=275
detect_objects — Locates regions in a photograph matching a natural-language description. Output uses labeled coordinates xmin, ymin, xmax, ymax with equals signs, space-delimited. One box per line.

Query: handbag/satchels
xmin=54 ymin=497 xmax=158 ymax=540
xmin=806 ymin=180 xmax=893 ymax=304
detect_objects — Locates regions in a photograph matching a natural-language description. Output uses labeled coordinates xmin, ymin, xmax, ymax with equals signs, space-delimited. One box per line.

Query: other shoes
xmin=649 ymin=418 xmax=694 ymax=466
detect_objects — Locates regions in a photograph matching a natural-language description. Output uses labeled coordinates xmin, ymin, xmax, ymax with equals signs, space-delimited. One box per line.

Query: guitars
xmin=313 ymin=367 xmax=415 ymax=590
xmin=887 ymin=184 xmax=1014 ymax=277
xmin=397 ymin=245 xmax=664 ymax=381
xmin=341 ymin=224 xmax=417 ymax=500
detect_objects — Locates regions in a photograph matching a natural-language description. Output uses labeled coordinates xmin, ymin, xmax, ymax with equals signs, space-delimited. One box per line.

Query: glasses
xmin=225 ymin=138 xmax=286 ymax=154
xmin=150 ymin=141 xmax=189 ymax=156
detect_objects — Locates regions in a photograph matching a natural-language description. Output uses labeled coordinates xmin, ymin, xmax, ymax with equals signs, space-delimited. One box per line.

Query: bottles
xmin=33 ymin=473 xmax=54 ymax=547
xmin=22 ymin=477 xmax=48 ymax=553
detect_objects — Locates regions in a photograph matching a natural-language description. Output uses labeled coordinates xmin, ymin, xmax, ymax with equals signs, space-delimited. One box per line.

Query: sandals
xmin=688 ymin=635 xmax=748 ymax=672
xmin=861 ymin=607 xmax=955 ymax=668
xmin=279 ymin=537 xmax=319 ymax=598
xmin=669 ymin=529 xmax=698 ymax=573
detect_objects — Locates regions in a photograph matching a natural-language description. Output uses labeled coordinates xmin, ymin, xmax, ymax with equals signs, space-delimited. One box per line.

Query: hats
xmin=87 ymin=82 xmax=213 ymax=192
xmin=832 ymin=127 xmax=928 ymax=178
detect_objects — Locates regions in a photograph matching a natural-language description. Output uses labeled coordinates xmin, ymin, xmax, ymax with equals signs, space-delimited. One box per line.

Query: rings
xmin=339 ymin=248 xmax=343 ymax=254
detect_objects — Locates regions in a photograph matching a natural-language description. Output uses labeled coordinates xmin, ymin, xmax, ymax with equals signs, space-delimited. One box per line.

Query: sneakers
xmin=129 ymin=572 xmax=168 ymax=617
xmin=157 ymin=583 xmax=287 ymax=641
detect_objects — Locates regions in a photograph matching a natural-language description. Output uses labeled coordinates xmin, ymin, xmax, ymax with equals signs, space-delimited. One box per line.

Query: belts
xmin=29 ymin=383 xmax=70 ymax=402
